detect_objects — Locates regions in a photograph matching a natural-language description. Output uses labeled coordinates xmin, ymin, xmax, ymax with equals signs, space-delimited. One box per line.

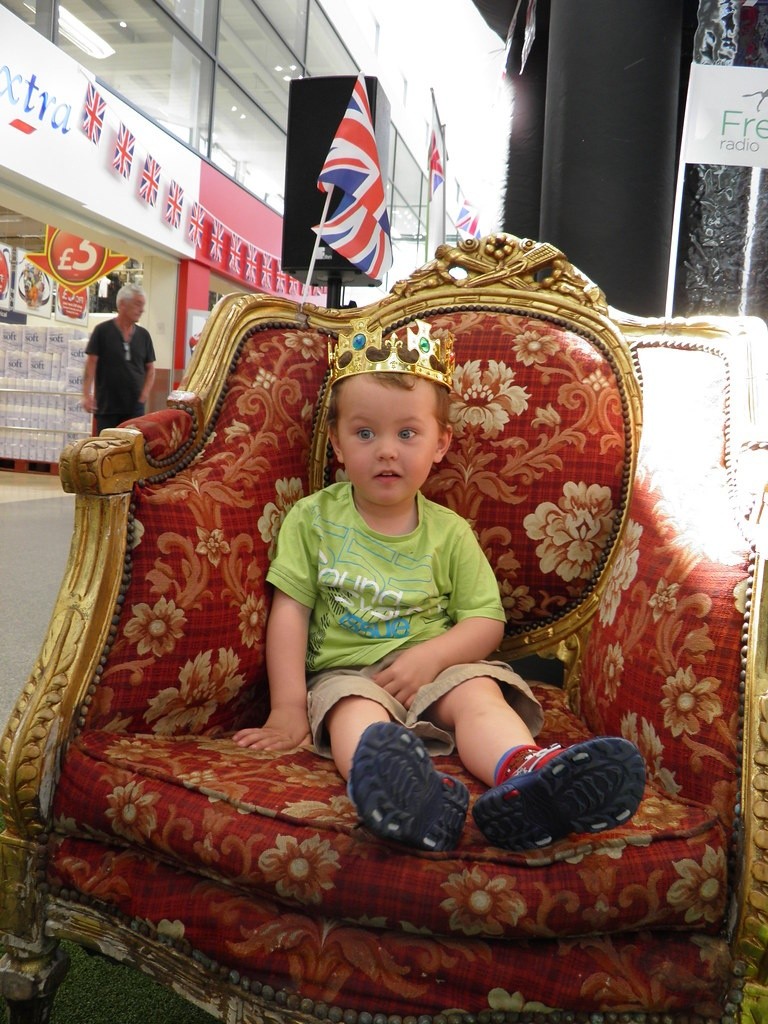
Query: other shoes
xmin=350 ymin=722 xmax=468 ymax=851
xmin=473 ymin=738 xmax=644 ymax=850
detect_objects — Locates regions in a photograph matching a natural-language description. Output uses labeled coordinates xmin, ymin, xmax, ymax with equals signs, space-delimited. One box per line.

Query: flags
xmin=456 ymin=199 xmax=481 ymax=239
xmin=188 ymin=201 xmax=205 ymax=249
xmin=275 ymin=260 xmax=286 ymax=292
xmin=311 ymin=72 xmax=394 ymax=279
xmin=138 ymin=153 xmax=162 ymax=207
xmin=289 ymin=275 xmax=318 ymax=296
xmin=209 ymin=219 xmax=224 ymax=262
xmin=80 ymin=82 xmax=108 ymax=145
xmin=228 ymin=234 xmax=242 ymax=274
xmin=426 ymin=128 xmax=443 ymax=202
xmin=111 ymin=120 xmax=135 ymax=180
xmin=260 ymin=253 xmax=272 ymax=290
xmin=165 ymin=179 xmax=186 ymax=228
xmin=244 ymin=244 xmax=257 ymax=283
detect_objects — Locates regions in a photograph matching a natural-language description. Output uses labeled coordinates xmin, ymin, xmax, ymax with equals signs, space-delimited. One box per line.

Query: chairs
xmin=0 ymin=234 xmax=768 ymax=1024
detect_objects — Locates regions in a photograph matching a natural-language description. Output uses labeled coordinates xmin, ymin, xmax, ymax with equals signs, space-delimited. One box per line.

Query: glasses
xmin=122 ymin=343 xmax=132 ymax=362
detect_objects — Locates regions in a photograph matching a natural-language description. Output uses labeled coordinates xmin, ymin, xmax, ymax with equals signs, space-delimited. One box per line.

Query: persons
xmin=83 ymin=285 xmax=157 ymax=436
xmin=232 ymin=318 xmax=649 ymax=856
